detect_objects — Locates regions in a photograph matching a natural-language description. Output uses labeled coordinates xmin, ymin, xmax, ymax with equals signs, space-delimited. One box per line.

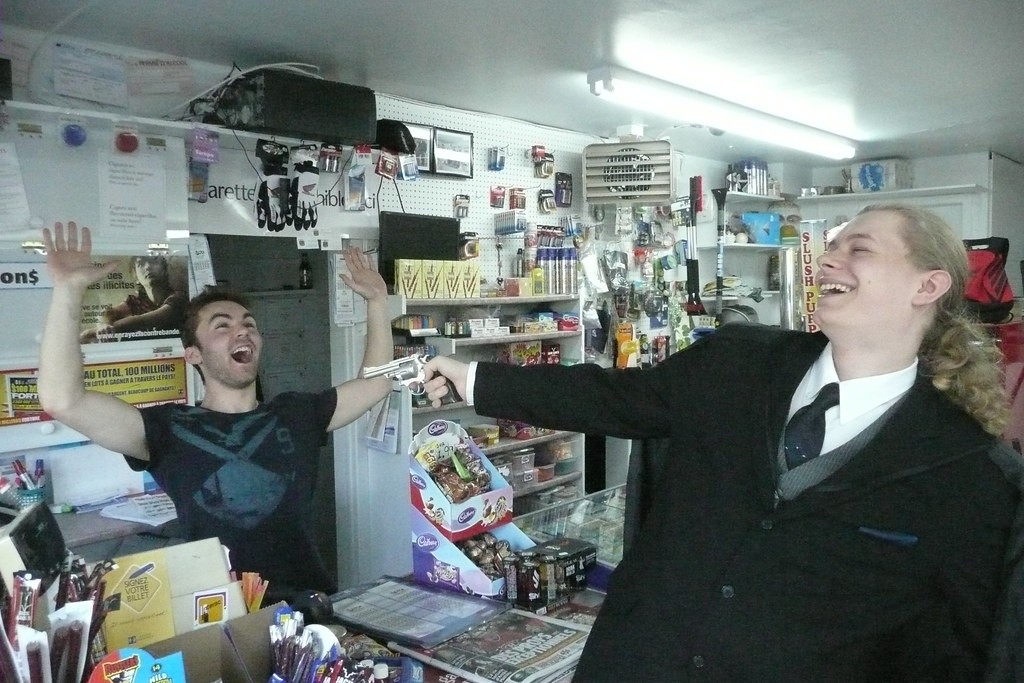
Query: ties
xmin=784 ymin=383 xmax=841 ymax=471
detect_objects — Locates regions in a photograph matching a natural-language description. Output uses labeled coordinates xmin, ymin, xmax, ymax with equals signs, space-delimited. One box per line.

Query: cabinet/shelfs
xmin=354 ymin=292 xmax=586 ymax=580
xmin=696 ymin=189 xmax=801 ymax=301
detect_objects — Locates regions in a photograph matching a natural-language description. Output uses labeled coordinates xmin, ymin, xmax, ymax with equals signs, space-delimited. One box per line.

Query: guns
xmin=360 ymin=352 xmax=463 ymax=406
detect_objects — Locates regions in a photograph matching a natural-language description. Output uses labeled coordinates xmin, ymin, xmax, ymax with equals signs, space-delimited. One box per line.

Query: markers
xmin=46 ymin=503 xmax=73 ymax=514
xmin=11 ymin=458 xmax=45 ymax=491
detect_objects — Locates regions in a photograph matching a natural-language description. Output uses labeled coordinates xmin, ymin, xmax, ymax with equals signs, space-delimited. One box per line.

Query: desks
xmin=50 ymin=486 xmax=165 ymax=549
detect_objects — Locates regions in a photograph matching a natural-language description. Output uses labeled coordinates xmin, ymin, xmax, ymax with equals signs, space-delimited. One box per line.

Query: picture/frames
xmin=400 ymin=120 xmax=475 ymax=179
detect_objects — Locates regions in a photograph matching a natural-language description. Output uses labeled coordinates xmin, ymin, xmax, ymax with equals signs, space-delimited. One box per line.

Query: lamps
xmin=588 ymin=65 xmax=859 ymax=160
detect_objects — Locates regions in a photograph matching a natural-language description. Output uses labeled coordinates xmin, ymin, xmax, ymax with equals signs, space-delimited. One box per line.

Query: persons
xmin=412 ymin=203 xmax=1024 ymax=683
xmin=78 ymin=256 xmax=185 ymax=344
xmin=37 ymin=221 xmax=394 ymax=603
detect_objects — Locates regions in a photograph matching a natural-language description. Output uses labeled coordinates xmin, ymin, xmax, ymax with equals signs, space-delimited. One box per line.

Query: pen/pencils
xmin=0 ymin=538 xmax=124 ymax=683
xmin=269 ymin=610 xmax=348 ymax=682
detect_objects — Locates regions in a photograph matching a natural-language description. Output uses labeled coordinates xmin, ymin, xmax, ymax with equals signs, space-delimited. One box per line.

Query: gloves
xmin=252 ymin=166 xmax=292 ymax=231
xmin=287 ymin=160 xmax=320 ymax=231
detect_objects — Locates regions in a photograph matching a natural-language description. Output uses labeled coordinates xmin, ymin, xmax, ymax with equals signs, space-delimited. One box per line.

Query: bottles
xmin=299 ymin=253 xmax=313 ymax=288
xmin=727 ymin=160 xmax=769 ymax=195
xmin=538 ymin=246 xmax=577 ymax=296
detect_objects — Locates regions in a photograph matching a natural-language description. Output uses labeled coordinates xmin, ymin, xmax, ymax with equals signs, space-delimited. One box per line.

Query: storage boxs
xmin=522 ymin=319 xmax=558 ymax=334
xmin=393 ymin=259 xmax=480 ymax=298
xmin=488 ymin=440 xmax=578 ymax=489
xmin=523 ymin=534 xmax=597 ymax=583
xmin=469 ymin=418 xmax=555 ymax=446
xmin=504 ymin=278 xmax=532 ymax=297
xmin=495 ymin=342 xmax=560 ymax=367
